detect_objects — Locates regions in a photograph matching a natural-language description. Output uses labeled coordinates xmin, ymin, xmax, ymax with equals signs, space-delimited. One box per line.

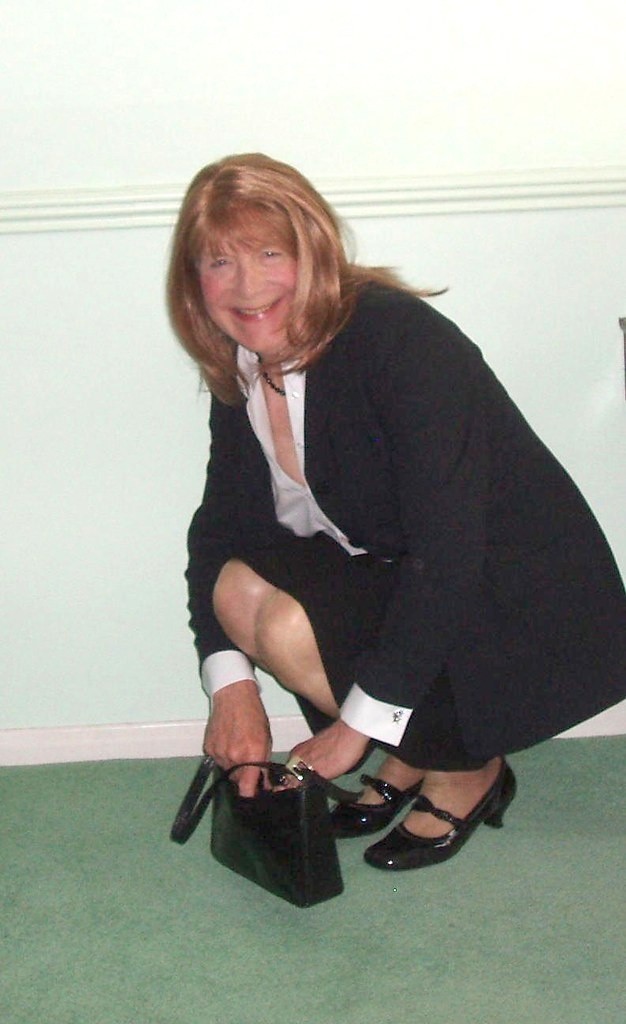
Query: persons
xmin=164 ymin=152 xmax=626 ymax=912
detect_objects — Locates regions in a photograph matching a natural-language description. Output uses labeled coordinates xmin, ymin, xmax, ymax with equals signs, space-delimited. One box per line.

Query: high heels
xmin=329 ymin=767 xmax=424 ymax=838
xmin=364 ymin=756 xmax=516 ymax=870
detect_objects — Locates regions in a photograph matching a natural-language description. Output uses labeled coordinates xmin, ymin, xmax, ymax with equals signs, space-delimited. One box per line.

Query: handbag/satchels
xmin=168 ymin=743 xmax=360 ymax=909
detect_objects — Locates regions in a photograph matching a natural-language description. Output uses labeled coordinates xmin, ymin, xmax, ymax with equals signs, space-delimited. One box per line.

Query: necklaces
xmin=252 ymin=351 xmax=288 ymax=398
